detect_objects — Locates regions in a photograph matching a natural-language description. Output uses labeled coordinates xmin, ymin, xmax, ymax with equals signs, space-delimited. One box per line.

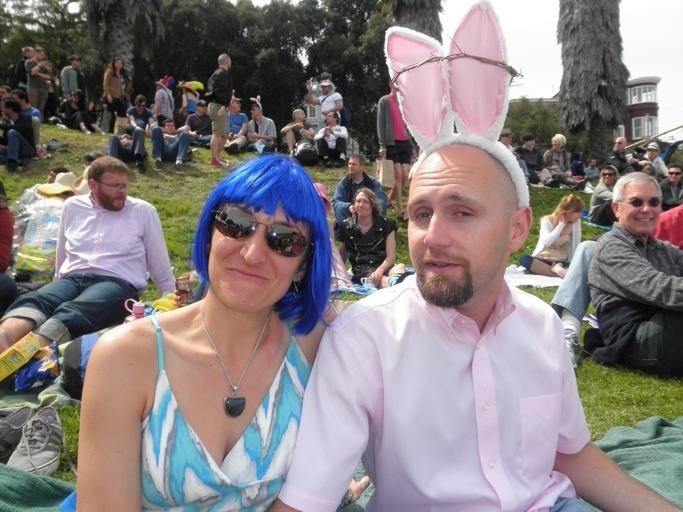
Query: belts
xmin=209 ymin=100 xmax=221 ymax=104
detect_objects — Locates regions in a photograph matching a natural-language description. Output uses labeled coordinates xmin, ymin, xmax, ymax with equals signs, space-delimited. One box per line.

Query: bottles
xmin=121 ymin=298 xmax=147 ymax=323
xmin=175 ymin=275 xmax=192 ymax=299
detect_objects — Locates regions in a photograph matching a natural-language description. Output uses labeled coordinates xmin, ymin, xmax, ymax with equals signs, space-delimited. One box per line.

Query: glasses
xmin=616 ymin=197 xmax=667 ymax=208
xmin=135 ymin=103 xmax=145 ymax=106
xmin=354 ymin=199 xmax=368 ymax=203
xmin=599 ymin=136 xmax=678 ymax=178
xmin=211 ymin=208 xmax=314 ymax=256
xmin=89 ymin=176 xmax=128 ymax=188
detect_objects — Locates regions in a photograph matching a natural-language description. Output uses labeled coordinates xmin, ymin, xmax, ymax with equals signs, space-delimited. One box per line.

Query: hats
xmin=72 ymin=165 xmax=90 ymax=195
xmin=229 ymin=98 xmax=241 ymax=103
xmin=37 ymin=172 xmax=76 ymax=196
xmin=68 ymin=55 xmax=81 ymax=61
xmin=195 ymin=99 xmax=206 ymax=105
xmin=309 ymin=183 xmax=331 ymax=215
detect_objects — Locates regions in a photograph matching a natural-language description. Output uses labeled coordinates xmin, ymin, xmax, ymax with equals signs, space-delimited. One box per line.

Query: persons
xmin=335 ymin=473 xmax=373 ymax=511
xmin=1 ymin=153 xmax=99 ymax=316
xmin=17 ymin=46 xmax=105 ymax=135
xmin=497 ymin=132 xmax=669 ymax=188
xmin=520 ymin=159 xmax=682 ymax=380
xmin=103 ymin=52 xmax=348 ymax=175
xmin=262 ymin=142 xmax=682 ymax=512
xmin=1 ymin=85 xmax=41 ymax=173
xmin=330 ymin=156 xmax=406 ymax=287
xmin=53 ymin=151 xmax=335 ymax=512
xmin=375 ymin=78 xmax=413 ymax=222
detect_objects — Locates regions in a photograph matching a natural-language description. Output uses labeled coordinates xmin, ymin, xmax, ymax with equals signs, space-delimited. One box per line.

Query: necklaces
xmin=193 ymin=307 xmax=272 ymax=417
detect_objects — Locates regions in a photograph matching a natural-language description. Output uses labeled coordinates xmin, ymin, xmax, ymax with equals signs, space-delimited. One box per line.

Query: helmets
xmin=296 ymin=141 xmax=316 ymax=168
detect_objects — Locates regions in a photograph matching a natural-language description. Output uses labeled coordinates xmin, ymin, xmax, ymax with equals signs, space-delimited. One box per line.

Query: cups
xmin=359 ymin=278 xmax=373 ymax=289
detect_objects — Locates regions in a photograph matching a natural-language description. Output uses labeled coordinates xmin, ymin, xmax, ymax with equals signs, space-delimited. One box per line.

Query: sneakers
xmin=7 ymin=406 xmax=67 ymax=475
xmin=173 ymin=162 xmax=183 ymax=175
xmin=210 ymin=157 xmax=230 ymax=171
xmin=135 ymin=158 xmax=146 ymax=174
xmin=563 ymin=330 xmax=581 ymax=374
xmin=323 ymin=158 xmax=346 ymax=168
xmin=0 ymin=405 xmax=34 ymax=464
xmin=153 ymin=159 xmax=161 ymax=170
